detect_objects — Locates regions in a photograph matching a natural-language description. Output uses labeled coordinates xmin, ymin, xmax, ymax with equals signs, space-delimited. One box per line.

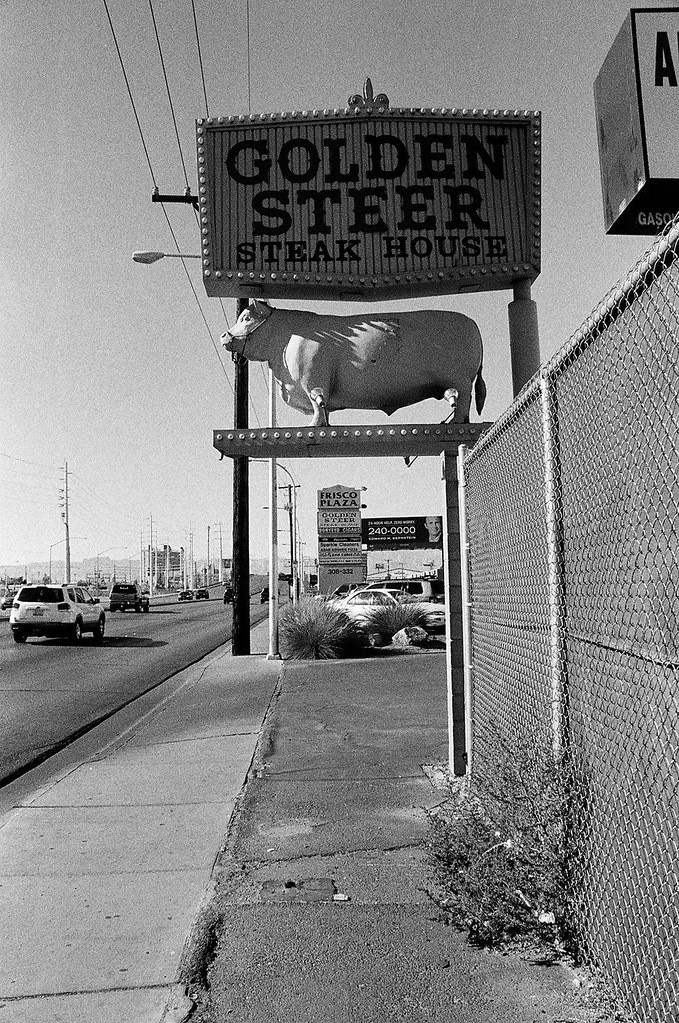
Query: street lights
xmin=96 ymin=546 xmax=128 ymax=586
xmin=131 ymin=250 xmax=250 ymax=657
xmin=248 ymin=459 xmax=295 ymax=606
xmin=49 ymin=537 xmax=87 ymax=584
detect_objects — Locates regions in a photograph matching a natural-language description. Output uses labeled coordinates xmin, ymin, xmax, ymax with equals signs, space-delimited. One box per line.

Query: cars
xmin=0 ymin=582 xmax=24 ymax=622
xmin=223 ymin=587 xmax=234 ymax=604
xmin=278 ymin=573 xmax=291 ymax=581
xmin=195 ymin=588 xmax=209 ymax=599
xmin=260 ymin=587 xmax=269 ymax=604
xmin=313 ymin=577 xmax=446 ymax=647
xmin=222 ymin=578 xmax=234 ymax=589
xmin=175 ymin=588 xmax=195 ymax=601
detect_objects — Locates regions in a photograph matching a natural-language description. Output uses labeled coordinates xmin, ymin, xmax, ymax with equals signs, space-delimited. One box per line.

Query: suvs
xmin=9 ymin=582 xmax=106 ymax=643
xmin=109 ymin=580 xmax=150 ymax=613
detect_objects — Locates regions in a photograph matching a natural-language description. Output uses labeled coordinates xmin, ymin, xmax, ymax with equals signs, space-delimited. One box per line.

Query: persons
xmin=424 ymin=516 xmax=442 ymax=542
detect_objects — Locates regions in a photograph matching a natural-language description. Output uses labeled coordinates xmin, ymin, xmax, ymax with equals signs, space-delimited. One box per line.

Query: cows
xmin=220 ymin=297 xmax=487 ymax=428
